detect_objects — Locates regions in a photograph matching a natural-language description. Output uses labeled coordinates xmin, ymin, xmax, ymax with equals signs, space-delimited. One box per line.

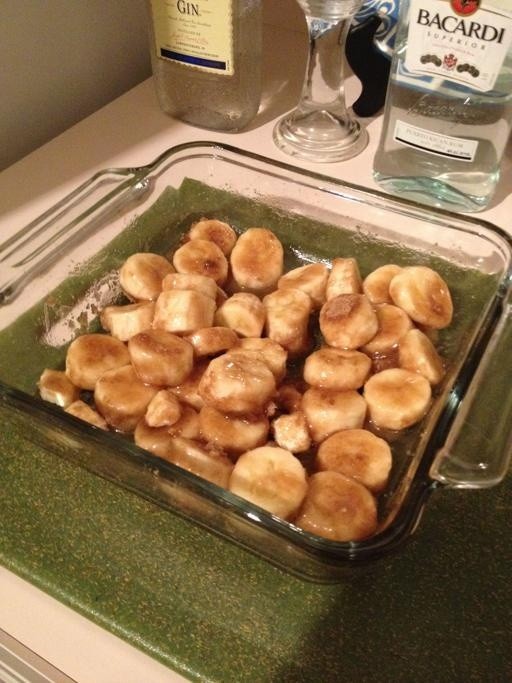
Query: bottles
xmin=371 ymin=0 xmax=511 ymax=213
xmin=145 ymin=0 xmax=266 ymax=132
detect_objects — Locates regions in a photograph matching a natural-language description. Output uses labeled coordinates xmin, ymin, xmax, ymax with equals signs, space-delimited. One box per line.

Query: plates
xmin=0 ymin=138 xmax=510 ymax=580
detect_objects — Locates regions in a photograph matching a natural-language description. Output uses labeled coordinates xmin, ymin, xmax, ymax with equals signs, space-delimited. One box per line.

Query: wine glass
xmin=272 ymin=0 xmax=371 ymax=162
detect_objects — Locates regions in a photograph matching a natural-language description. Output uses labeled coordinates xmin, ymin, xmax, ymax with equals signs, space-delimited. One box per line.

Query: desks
xmin=0 ymin=23 xmax=512 ymax=682
